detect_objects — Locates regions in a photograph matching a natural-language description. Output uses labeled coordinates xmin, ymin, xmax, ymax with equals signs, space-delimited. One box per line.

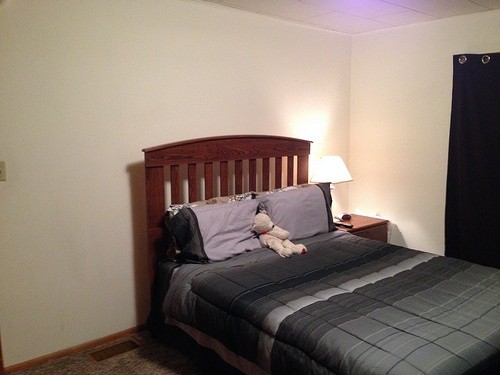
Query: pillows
xmin=251 ymin=184 xmax=309 ymax=213
xmin=255 ymin=182 xmax=339 ymax=240
xmin=171 ymin=199 xmax=263 ymax=263
xmin=165 ymin=192 xmax=250 ymax=261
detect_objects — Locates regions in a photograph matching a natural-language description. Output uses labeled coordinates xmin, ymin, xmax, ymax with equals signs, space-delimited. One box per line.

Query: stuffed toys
xmin=251 ymin=212 xmax=308 ymax=258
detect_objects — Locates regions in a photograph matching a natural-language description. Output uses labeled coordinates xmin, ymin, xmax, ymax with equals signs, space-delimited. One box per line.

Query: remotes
xmin=335 ymin=221 xmax=353 ymax=228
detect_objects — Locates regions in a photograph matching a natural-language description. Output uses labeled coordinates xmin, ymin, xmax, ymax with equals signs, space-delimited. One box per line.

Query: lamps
xmin=309 ymin=155 xmax=353 ymax=209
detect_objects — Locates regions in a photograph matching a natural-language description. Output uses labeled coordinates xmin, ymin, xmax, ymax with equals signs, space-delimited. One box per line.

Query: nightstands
xmin=336 ymin=214 xmax=390 ymax=243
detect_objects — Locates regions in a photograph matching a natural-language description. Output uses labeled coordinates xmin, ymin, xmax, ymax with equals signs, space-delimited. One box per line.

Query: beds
xmin=142 ymin=134 xmax=500 ymax=375
